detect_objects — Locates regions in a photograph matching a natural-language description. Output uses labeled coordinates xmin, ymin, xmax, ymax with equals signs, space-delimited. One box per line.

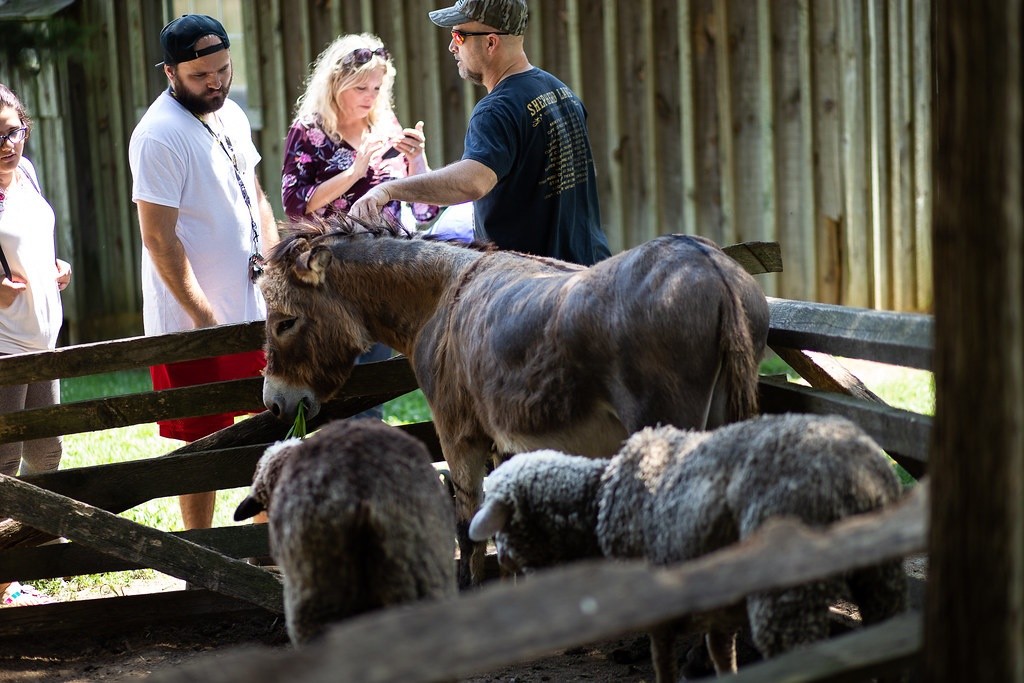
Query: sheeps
xmin=467 ymin=410 xmax=908 ymax=682
xmin=233 ymin=417 xmax=456 ymax=647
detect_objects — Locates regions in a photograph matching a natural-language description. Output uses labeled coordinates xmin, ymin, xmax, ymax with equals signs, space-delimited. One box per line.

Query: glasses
xmin=340 ymin=48 xmax=389 ymax=75
xmin=0 ymin=120 xmax=29 ymax=147
xmin=451 ymin=29 xmax=509 ymax=45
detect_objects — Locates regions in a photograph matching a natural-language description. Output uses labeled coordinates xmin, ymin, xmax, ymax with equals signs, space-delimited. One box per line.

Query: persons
xmin=280 ymin=30 xmax=438 ymax=419
xmin=131 ymin=14 xmax=282 ymax=590
xmin=344 ymin=0 xmax=615 ymax=269
xmin=0 ymin=84 xmax=73 ymax=479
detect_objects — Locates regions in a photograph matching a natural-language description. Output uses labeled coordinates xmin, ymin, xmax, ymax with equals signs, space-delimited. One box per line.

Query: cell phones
xmin=381 ymin=135 xmax=414 ymax=159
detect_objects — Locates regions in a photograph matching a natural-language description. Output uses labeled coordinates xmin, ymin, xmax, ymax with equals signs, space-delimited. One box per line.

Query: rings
xmin=410 ymin=147 xmax=416 ymax=153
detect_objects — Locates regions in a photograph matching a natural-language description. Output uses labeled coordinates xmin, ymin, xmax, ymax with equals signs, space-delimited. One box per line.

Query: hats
xmin=428 ymin=0 xmax=527 ymax=33
xmin=154 ymin=15 xmax=230 ymax=68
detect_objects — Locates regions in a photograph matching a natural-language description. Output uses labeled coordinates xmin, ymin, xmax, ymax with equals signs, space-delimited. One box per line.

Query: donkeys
xmin=261 ymin=211 xmax=769 ymax=585
